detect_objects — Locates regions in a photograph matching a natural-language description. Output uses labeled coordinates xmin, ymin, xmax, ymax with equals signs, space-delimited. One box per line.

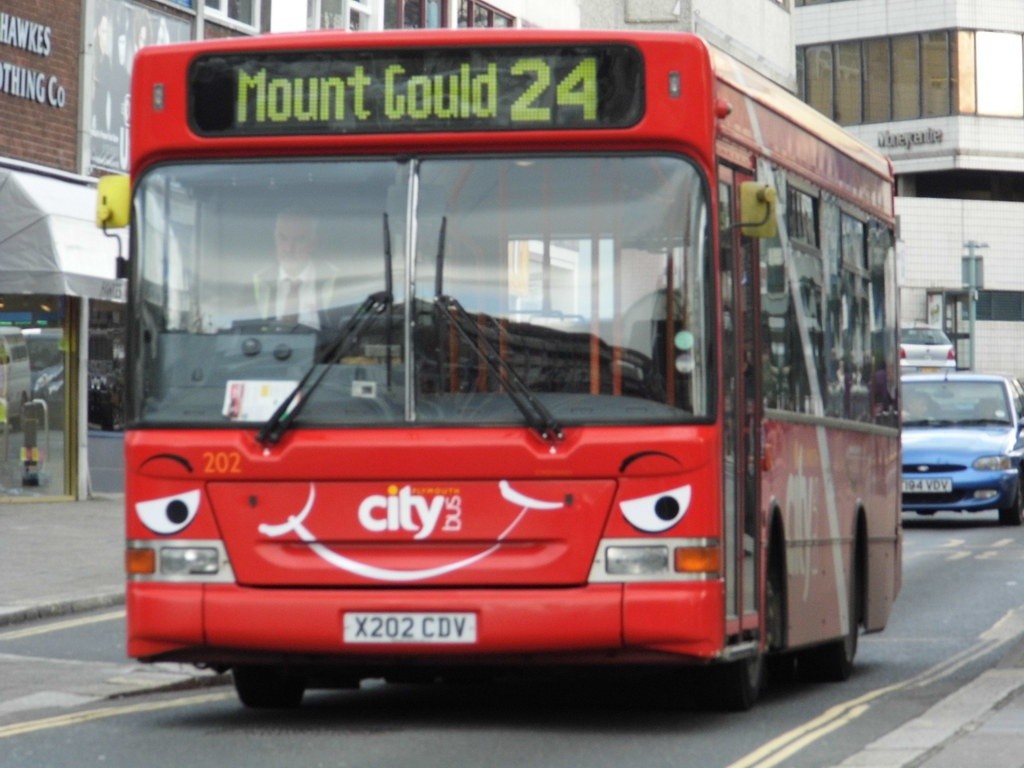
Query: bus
xmin=95 ymin=28 xmax=906 ymax=722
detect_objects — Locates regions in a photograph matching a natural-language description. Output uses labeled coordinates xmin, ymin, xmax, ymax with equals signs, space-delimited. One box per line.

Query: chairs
xmin=974 ymin=396 xmax=1001 ymax=419
xmin=618 ymin=290 xmax=686 ymax=401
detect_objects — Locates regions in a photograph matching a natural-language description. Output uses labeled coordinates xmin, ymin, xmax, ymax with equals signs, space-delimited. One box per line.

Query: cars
xmin=0 ymin=322 xmax=176 ymax=436
xmin=893 ymin=371 xmax=1024 ymax=528
xmin=869 ymin=323 xmax=958 ymax=379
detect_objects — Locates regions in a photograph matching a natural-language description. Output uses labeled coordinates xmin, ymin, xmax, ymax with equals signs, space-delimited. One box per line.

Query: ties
xmin=281 ymin=278 xmax=304 ymax=324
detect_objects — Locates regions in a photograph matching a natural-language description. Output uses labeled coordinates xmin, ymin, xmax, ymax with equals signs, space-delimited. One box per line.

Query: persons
xmin=226 ymin=206 xmax=362 ymax=362
xmin=91 ymin=12 xmax=171 ymax=134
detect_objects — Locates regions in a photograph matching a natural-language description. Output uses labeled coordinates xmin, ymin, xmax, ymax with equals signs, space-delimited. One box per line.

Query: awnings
xmin=0 ymin=167 xmax=128 ymax=306
